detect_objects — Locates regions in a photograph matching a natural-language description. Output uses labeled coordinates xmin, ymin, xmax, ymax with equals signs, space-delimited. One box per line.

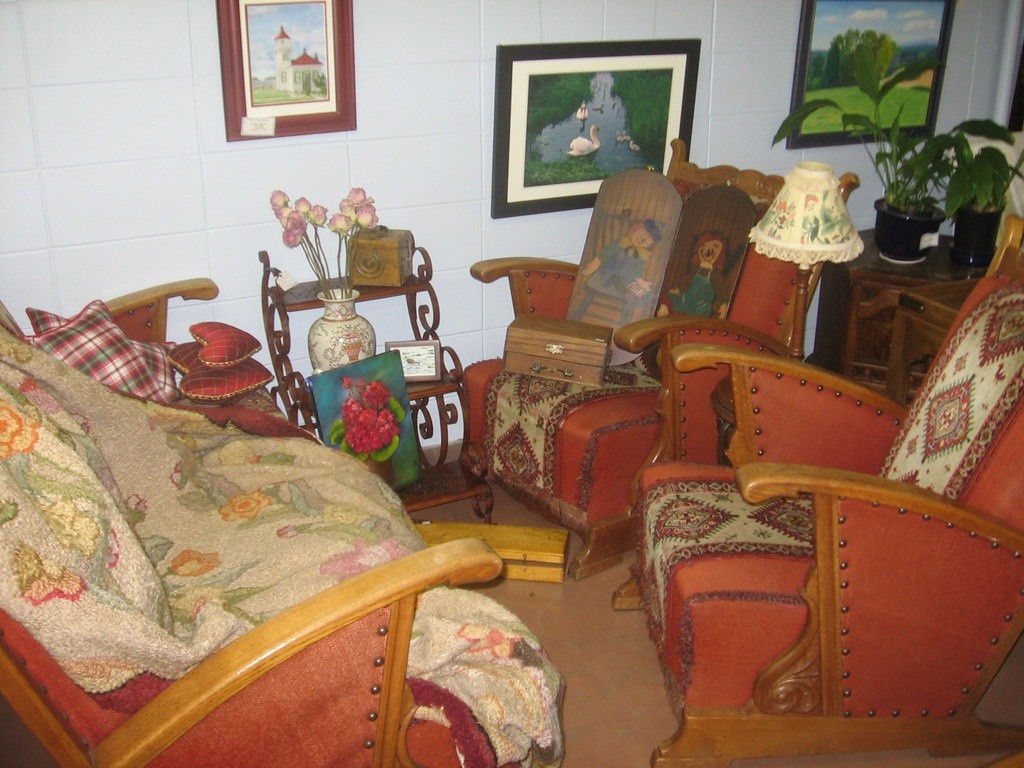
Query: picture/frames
xmin=786 ymin=0 xmax=957 ymax=151
xmin=216 ymin=0 xmax=357 ymax=143
xmin=386 ymin=338 xmax=443 ymax=383
xmin=491 ymin=38 xmax=701 ymax=218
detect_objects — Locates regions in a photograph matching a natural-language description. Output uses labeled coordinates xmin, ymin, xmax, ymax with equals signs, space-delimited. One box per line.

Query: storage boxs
xmin=415 ymin=520 xmax=571 ymax=584
xmin=504 ymin=316 xmax=614 ymax=391
xmin=345 ymin=224 xmax=413 ymax=287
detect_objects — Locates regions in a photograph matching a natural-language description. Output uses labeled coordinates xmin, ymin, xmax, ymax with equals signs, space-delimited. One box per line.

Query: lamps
xmin=750 ymin=162 xmax=866 ymax=363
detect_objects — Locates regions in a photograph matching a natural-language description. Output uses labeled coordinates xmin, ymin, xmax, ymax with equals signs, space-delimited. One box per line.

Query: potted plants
xmin=768 ymin=33 xmax=1016 ymax=261
xmin=945 ymin=132 xmax=1024 ymax=253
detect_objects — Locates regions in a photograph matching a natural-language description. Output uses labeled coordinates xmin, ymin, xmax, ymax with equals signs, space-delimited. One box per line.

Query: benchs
xmin=0 ymin=277 xmax=567 ymax=768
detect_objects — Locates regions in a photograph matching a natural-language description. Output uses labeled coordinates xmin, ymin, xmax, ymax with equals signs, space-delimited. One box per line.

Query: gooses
xmin=616 ymin=130 xmax=641 ymax=152
xmin=567 ymin=125 xmax=601 ymax=157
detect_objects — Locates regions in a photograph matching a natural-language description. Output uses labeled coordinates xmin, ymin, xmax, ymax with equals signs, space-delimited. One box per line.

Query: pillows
xmin=32 ymin=300 xmax=172 ymax=406
xmin=168 ymin=341 xmax=276 ymax=406
xmin=26 ymin=308 xmax=181 ymax=400
xmin=167 ymin=404 xmax=324 ymax=445
xmin=188 ymin=320 xmax=264 ymax=369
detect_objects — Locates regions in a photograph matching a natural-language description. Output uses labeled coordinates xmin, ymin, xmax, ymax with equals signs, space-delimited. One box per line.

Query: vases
xmin=307 ymin=288 xmax=375 ymax=372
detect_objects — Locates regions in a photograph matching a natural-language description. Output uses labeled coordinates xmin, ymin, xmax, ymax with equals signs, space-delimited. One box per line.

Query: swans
xmin=576 ymin=99 xmax=588 ymax=120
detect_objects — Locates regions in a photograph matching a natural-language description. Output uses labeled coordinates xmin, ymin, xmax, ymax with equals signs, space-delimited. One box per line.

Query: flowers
xmin=265 ymin=186 xmax=378 ymax=296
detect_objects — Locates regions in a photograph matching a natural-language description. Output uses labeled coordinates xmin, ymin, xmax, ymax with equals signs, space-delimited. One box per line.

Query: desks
xmin=885 ymin=275 xmax=980 ymax=407
xmin=839 ymin=228 xmax=986 ymax=383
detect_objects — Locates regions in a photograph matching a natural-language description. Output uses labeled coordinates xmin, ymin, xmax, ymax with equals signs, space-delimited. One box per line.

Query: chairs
xmin=612 ymin=273 xmax=1024 ymax=768
xmin=461 ymin=138 xmax=861 ymax=580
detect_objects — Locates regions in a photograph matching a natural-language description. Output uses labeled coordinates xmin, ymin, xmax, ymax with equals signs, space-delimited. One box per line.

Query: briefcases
xmin=502 ymin=313 xmax=614 ymax=386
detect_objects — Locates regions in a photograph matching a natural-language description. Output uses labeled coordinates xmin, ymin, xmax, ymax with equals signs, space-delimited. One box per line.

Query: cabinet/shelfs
xmin=258 ymin=246 xmax=497 ymax=525
